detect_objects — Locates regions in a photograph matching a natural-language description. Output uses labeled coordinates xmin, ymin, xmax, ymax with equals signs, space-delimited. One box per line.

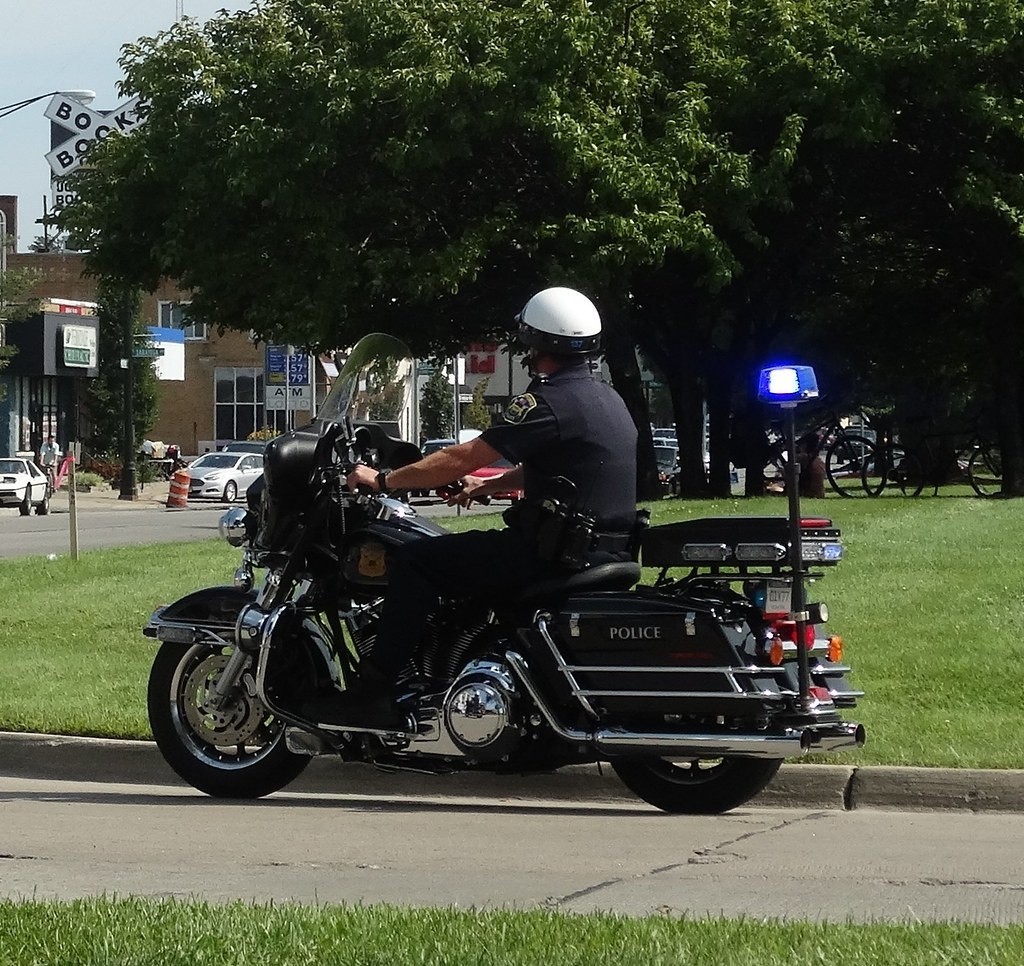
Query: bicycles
xmin=756 ymin=382 xmax=1003 ymax=499
xmin=41 ymin=465 xmax=55 ymax=497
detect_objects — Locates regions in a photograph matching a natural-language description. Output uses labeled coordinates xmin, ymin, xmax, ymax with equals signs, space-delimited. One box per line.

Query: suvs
xmin=838 ymin=429 xmax=877 ymax=463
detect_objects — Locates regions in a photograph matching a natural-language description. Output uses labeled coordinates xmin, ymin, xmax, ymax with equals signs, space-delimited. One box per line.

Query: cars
xmin=419 ymin=437 xmax=457 ymax=496
xmin=222 ymin=440 xmax=265 ymax=455
xmin=0 ymin=456 xmax=50 ymax=516
xmin=169 ymin=451 xmax=264 ymax=503
xmin=651 ymin=422 xmax=837 ymax=493
xmin=469 ymin=458 xmax=524 ymax=505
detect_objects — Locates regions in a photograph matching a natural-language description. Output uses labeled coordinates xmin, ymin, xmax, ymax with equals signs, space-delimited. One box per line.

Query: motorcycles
xmin=143 ymin=333 xmax=866 ymax=815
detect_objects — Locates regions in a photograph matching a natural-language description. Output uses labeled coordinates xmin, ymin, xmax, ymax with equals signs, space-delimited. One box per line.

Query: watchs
xmin=378 ymin=468 xmax=391 ymax=493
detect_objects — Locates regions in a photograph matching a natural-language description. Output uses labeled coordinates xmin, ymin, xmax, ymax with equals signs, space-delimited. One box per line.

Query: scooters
xmin=167 ymin=444 xmax=192 ymax=477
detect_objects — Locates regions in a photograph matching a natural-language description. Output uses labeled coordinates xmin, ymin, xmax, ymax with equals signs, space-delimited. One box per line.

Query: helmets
xmin=514 ymin=286 xmax=603 ymax=365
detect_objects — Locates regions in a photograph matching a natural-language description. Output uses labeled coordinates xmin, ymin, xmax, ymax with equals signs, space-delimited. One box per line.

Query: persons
xmin=39 ymin=435 xmax=60 ymax=493
xmin=348 ymin=286 xmax=638 ymax=739
xmin=795 ymin=432 xmax=825 ymax=499
xmin=164 ymin=446 xmax=182 ymax=476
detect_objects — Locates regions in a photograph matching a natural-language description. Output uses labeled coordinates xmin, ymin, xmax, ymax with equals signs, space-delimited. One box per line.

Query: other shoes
xmin=303 ymin=689 xmax=406 ymax=729
